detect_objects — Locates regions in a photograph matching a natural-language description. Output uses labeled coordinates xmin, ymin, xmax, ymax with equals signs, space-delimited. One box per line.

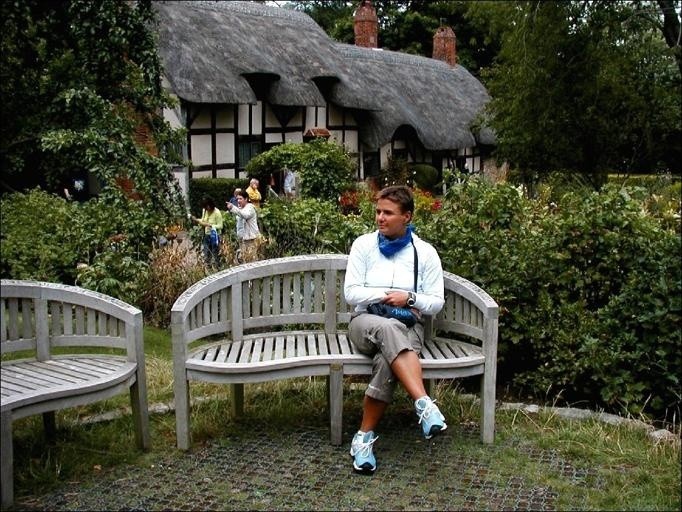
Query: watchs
xmin=407 ymin=290 xmax=415 ymax=308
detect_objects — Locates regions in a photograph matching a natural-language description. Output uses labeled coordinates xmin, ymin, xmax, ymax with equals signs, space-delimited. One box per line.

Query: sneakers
xmin=350 ymin=431 xmax=379 ymax=472
xmin=414 ymin=396 xmax=447 ymax=440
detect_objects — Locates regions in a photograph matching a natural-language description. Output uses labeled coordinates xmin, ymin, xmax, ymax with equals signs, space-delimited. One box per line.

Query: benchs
xmin=170 ymin=254 xmax=497 ymax=452
xmin=0 ymin=279 xmax=152 ymax=510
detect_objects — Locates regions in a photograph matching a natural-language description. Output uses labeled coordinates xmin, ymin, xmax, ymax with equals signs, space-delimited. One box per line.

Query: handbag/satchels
xmin=367 ymin=303 xmax=417 ymax=328
xmin=210 ymin=224 xmax=218 ymax=248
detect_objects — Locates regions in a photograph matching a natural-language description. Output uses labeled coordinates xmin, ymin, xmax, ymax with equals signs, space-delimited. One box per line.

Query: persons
xmin=245 ymin=177 xmax=261 ymax=208
xmin=284 ymin=169 xmax=296 ymax=201
xmin=228 ymin=188 xmax=243 ymax=213
xmin=191 ymin=194 xmax=223 ymax=270
xmin=225 ymin=192 xmax=260 ymax=262
xmin=343 ymin=186 xmax=448 ymax=476
